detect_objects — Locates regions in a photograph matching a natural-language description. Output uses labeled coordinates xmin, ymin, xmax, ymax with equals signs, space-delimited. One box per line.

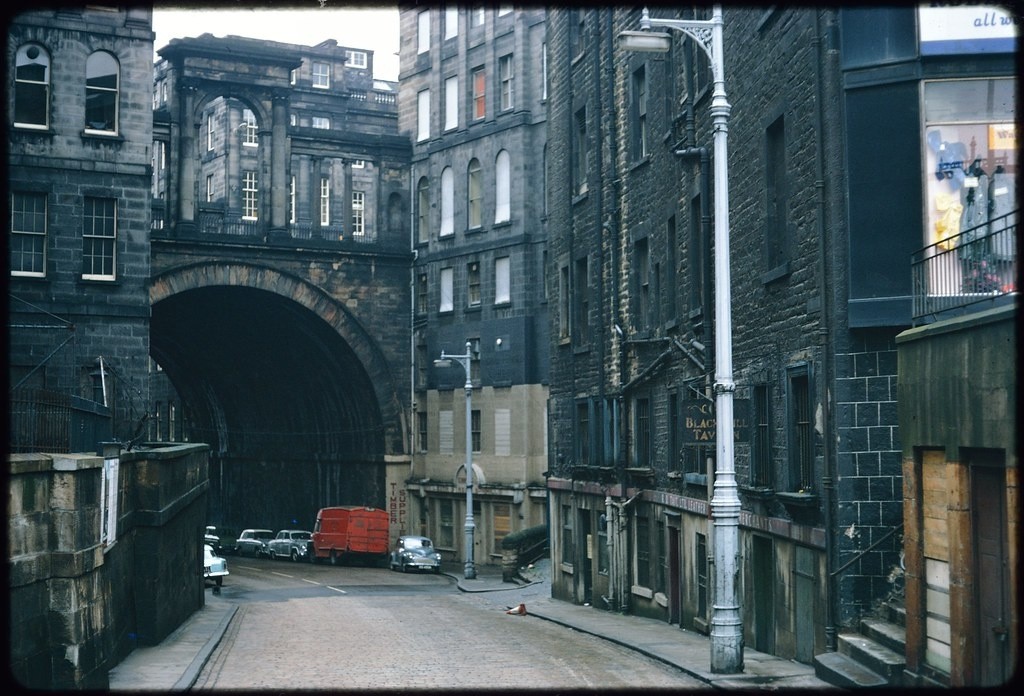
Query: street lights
xmin=432 ymin=342 xmax=478 ymax=579
xmin=618 ymin=3 xmax=746 ymax=674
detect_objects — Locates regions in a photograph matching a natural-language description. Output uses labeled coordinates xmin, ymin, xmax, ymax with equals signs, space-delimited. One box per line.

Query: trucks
xmin=309 ymin=505 xmax=390 ymax=566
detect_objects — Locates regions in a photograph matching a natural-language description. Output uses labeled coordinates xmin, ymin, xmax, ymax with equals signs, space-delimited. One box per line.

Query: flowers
xmin=959 ymin=250 xmax=1003 ymax=293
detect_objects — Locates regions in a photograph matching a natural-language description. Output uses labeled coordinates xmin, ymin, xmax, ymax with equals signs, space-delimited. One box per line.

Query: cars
xmin=236 ymin=529 xmax=275 ymax=557
xmin=388 ymin=536 xmax=442 ymax=573
xmin=203 ymin=545 xmax=229 ymax=586
xmin=205 ymin=526 xmax=220 ymax=551
xmin=267 ymin=530 xmax=313 ymax=563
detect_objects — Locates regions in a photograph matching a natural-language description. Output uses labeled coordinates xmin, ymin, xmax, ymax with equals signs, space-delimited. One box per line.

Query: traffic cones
xmin=506 ymin=603 xmax=527 ymax=617
